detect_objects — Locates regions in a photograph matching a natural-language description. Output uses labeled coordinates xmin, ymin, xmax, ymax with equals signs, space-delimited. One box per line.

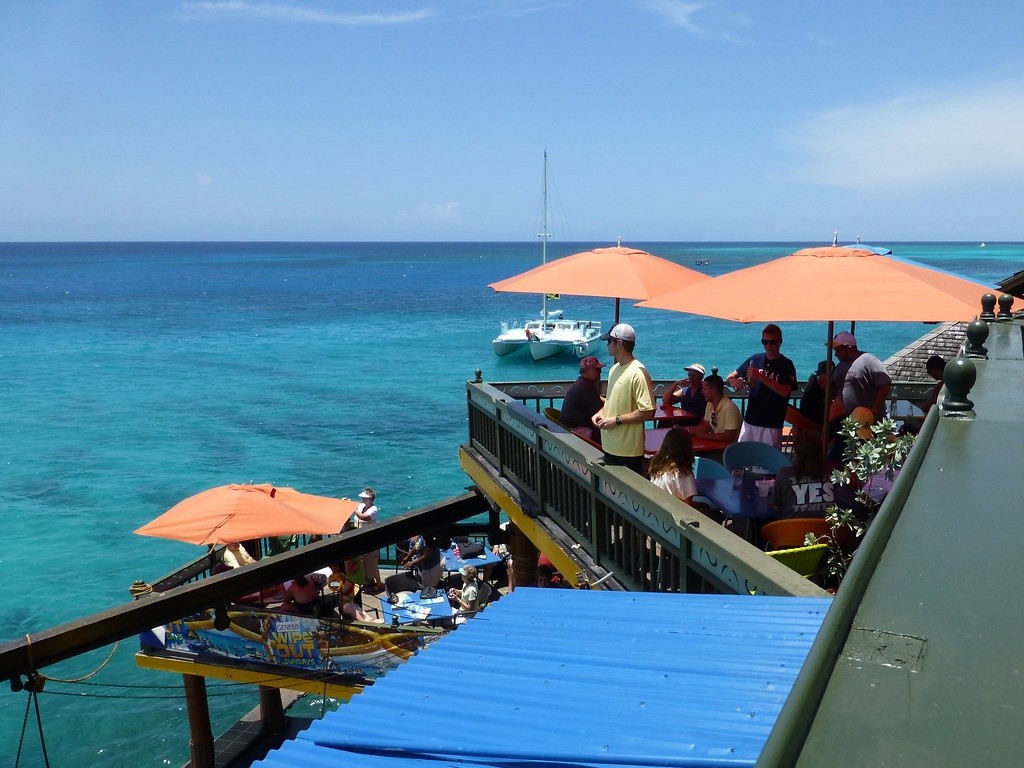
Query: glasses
xmin=687 ymin=372 xmax=701 ymax=376
xmin=711 ymin=411 xmax=717 ymax=427
xmin=608 ymin=339 xmax=618 ymax=344
xmin=761 ymin=336 xmax=781 ymax=345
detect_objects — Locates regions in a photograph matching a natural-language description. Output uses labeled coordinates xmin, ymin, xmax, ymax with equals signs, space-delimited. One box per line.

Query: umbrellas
xmin=633 ymin=232 xmax=1024 ymax=477
xmin=486 ymin=237 xmax=715 ymax=363
xmin=844 ymin=234 xmax=1004 ymax=335
xmin=131 ymin=481 xmax=361 ymax=553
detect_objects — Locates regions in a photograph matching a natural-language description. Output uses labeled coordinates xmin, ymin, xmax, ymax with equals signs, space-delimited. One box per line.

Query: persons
xmin=525 ymin=328 xmax=531 ymax=340
xmin=591 ymin=323 xmax=656 ymax=558
xmin=481 ymin=516 xmax=572 ymax=593
xmin=657 ymin=363 xmax=742 ymax=441
xmin=646 ymin=427 xmax=698 ymax=559
xmin=800 ymin=331 xmax=946 ymax=509
xmin=223 ymin=540 xmax=255 ymax=568
xmin=341 ymin=488 xmax=382 ymax=593
xmin=444 ymin=565 xmax=480 ymax=625
xmin=279 ymin=574 xmax=320 ymax=614
xmin=561 ymin=356 xmax=607 ymax=444
xmin=559 ymin=314 xmax=564 ymax=319
xmin=402 ymin=536 xmax=446 ymax=589
xmin=725 ymin=322 xmax=800 ymax=451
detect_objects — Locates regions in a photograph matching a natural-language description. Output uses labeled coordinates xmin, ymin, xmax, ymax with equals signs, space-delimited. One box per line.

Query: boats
xmin=695 ymin=259 xmax=709 ymax=266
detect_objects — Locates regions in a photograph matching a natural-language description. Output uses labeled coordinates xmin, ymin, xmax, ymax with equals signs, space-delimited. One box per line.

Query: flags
xmin=546 ymin=294 xmax=561 ymax=300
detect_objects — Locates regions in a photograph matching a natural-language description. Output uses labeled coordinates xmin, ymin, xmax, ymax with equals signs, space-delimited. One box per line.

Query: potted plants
xmin=830 ymin=416 xmax=918 ymax=526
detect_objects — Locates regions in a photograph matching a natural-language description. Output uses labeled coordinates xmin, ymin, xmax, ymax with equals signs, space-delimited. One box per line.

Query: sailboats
xmin=492 ymin=146 xmax=603 ymax=361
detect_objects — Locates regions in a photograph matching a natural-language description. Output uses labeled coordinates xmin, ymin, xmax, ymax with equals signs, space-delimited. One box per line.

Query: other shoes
xmin=372 ymin=582 xmax=384 ymax=591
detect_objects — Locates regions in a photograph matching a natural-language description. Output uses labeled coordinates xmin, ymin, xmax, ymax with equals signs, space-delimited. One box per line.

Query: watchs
xmin=615 ymin=415 xmax=622 ymax=425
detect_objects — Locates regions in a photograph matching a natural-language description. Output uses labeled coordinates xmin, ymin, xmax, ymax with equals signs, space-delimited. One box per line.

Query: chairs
xmin=543 ymin=405 xmax=837 ymax=578
xmin=214 ymin=538 xmax=514 ymax=630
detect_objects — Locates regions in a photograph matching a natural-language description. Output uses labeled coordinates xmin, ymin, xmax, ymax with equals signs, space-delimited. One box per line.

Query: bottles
xmin=890 ymin=386 xmax=898 ymax=408
xmin=745 ymin=359 xmax=755 ymax=390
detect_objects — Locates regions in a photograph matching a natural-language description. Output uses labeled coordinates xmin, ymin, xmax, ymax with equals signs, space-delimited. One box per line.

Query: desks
xmin=638 ymin=425 xmax=732 ymax=455
xmin=381 ymin=587 xmax=452 ymax=629
xmin=437 ymin=545 xmax=502 ymax=586
xmin=694 ymin=474 xmax=847 ymax=541
xmin=643 ymin=402 xmax=694 ymax=428
xmin=882 ymin=397 xmax=926 ymax=432
xmin=237 ymin=572 xmax=328 ymax=609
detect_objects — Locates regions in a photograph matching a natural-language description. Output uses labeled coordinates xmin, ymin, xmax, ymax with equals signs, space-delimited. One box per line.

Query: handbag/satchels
xmin=457 ymin=543 xmax=486 ymax=559
xmin=741 ymin=471 xmax=776 ymax=496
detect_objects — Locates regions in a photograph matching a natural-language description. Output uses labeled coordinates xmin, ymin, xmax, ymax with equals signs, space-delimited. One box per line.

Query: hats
xmin=815 ymin=360 xmax=836 ymax=374
xmin=850 ymin=407 xmax=874 ymax=439
xmin=824 ymin=331 xmax=856 ymax=349
xmin=579 ymin=356 xmax=606 ymax=369
xmin=684 ymin=364 xmax=706 ymax=375
xmin=358 ymin=491 xmax=374 ymax=499
xmin=600 ymin=323 xmax=636 ymax=341
xmin=339 ymin=581 xmax=360 ymax=603
xmin=459 ymin=565 xmax=477 ymax=580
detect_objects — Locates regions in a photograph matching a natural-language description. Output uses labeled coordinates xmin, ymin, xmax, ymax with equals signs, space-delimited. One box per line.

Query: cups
xmin=731 ymin=469 xmax=745 ymax=484
xmin=754 ymin=480 xmax=773 ymax=497
